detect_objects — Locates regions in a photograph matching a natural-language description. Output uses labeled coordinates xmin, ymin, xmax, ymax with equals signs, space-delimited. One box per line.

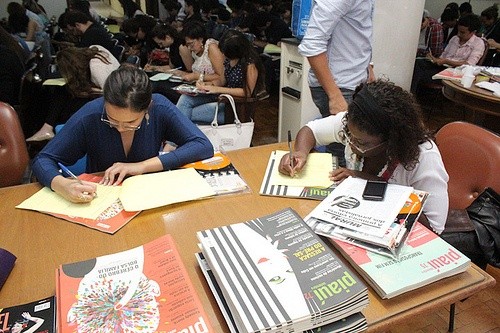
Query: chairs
xmin=0 ymin=101 xmax=29 ymax=188
xmin=433 ymin=120 xmax=500 ymax=333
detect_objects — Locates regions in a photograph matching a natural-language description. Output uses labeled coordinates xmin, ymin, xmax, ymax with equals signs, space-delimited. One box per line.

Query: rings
xmin=81 ymin=196 xmax=86 ymax=200
xmin=104 ymin=177 xmax=109 ymax=180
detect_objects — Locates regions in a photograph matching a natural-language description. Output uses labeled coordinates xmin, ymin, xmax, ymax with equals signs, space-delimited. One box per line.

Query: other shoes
xmin=26 ymin=132 xmax=55 ymax=142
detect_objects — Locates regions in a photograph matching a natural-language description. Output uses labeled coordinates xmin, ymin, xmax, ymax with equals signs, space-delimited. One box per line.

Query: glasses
xmin=342 ymin=125 xmax=387 ymax=155
xmin=100 ymin=106 xmax=145 ymax=130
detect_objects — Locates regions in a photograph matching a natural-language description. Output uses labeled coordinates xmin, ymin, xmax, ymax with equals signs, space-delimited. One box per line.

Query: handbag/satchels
xmin=150 ymin=48 xmax=170 ymax=63
xmin=198 ymin=94 xmax=255 ymax=152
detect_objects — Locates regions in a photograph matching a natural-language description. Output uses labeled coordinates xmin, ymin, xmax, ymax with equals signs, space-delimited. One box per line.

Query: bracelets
xmin=229 ymin=87 xmax=232 ymax=95
xmin=369 ymin=62 xmax=374 ymax=68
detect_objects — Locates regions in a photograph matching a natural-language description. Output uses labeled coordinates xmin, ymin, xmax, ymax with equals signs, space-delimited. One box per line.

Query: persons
xmin=414 ymin=2 xmax=500 ymax=85
xmin=31 ymin=62 xmax=214 ymax=204
xmin=298 ymin=0 xmax=376 ymax=118
xmin=278 ymin=72 xmax=450 ymax=236
xmin=0 ymin=0 xmax=292 ymax=142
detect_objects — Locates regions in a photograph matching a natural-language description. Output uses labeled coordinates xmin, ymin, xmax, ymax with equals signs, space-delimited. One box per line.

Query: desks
xmin=1 ymin=140 xmax=496 ymax=333
xmin=436 ymin=79 xmax=500 ymax=131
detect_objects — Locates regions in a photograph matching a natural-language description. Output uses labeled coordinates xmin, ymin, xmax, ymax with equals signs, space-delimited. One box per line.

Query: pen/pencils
xmin=174 ymin=67 xmax=182 ymax=70
xmin=58 ymin=162 xmax=98 ymax=198
xmin=288 ymin=130 xmax=293 ymax=168
xmin=203 ymin=70 xmax=205 ymax=81
xmin=147 ymin=59 xmax=151 ymax=64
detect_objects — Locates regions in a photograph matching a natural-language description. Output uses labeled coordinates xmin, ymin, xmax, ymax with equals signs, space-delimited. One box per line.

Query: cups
xmin=464 ymin=75 xmax=475 ymax=87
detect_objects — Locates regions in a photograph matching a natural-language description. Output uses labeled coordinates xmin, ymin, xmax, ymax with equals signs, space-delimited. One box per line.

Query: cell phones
xmin=362 ymin=181 xmax=387 ymax=201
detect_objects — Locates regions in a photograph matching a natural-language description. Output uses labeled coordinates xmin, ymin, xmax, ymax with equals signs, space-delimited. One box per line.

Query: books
xmin=432 ymin=65 xmax=500 ymax=97
xmin=4 ymin=148 xmax=471 ymax=333
